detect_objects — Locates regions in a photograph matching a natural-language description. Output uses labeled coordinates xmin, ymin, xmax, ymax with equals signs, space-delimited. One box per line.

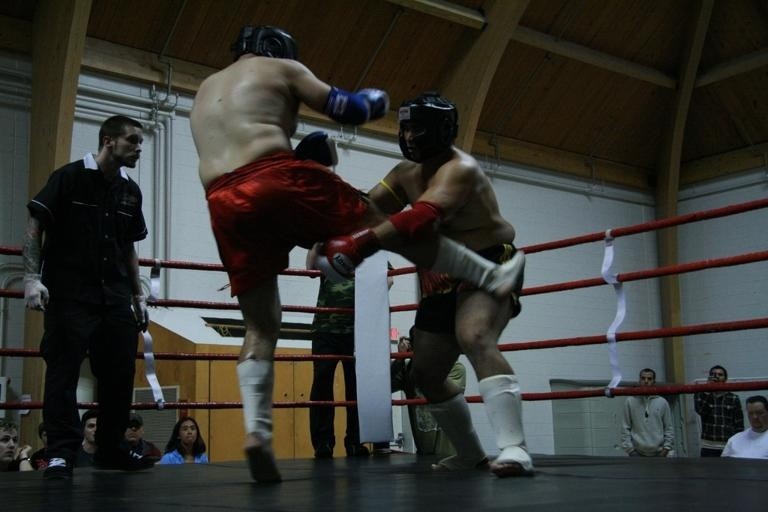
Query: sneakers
xmin=42 ymin=457 xmax=74 ymax=481
xmin=315 ymin=443 xmax=333 ymax=458
xmin=490 ymin=446 xmax=536 ymax=477
xmin=346 ymin=444 xmax=369 ymax=459
xmin=91 ymin=449 xmax=154 ymax=470
xmin=431 ymin=454 xmax=490 ymax=472
xmin=488 ymin=249 xmax=525 ymax=299
xmin=245 ymin=433 xmax=280 ymax=483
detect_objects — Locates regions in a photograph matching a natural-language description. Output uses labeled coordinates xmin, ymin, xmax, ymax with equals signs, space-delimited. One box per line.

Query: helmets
xmin=399 ymin=92 xmax=459 ymax=163
xmin=230 ymin=24 xmax=298 ymax=60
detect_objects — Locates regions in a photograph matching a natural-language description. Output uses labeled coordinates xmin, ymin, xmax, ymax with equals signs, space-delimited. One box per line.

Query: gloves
xmin=23 ymin=274 xmax=52 ymax=312
xmin=132 ymin=293 xmax=149 ymax=331
xmin=293 ymin=131 xmax=338 ymax=167
xmin=355 ymin=89 xmax=391 ymax=122
xmin=305 ymin=228 xmax=381 ymax=285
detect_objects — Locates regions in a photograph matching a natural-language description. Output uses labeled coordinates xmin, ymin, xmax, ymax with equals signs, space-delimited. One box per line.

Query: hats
xmin=127 ymin=412 xmax=144 ymax=426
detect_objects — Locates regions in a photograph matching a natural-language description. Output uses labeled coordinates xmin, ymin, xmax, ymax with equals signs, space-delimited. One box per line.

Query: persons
xmin=721 ymin=396 xmax=767 ymax=459
xmin=190 ymin=22 xmax=527 ymax=483
xmin=693 ymin=366 xmax=744 ymax=458
xmin=389 ymin=323 xmax=466 ymax=462
xmin=306 ymin=89 xmax=539 ymax=478
xmin=303 ymin=230 xmax=395 ymax=461
xmin=20 ymin=115 xmax=154 ymax=476
xmin=1 ymin=412 xmax=212 ymax=474
xmin=622 ymin=368 xmax=674 ymax=458
